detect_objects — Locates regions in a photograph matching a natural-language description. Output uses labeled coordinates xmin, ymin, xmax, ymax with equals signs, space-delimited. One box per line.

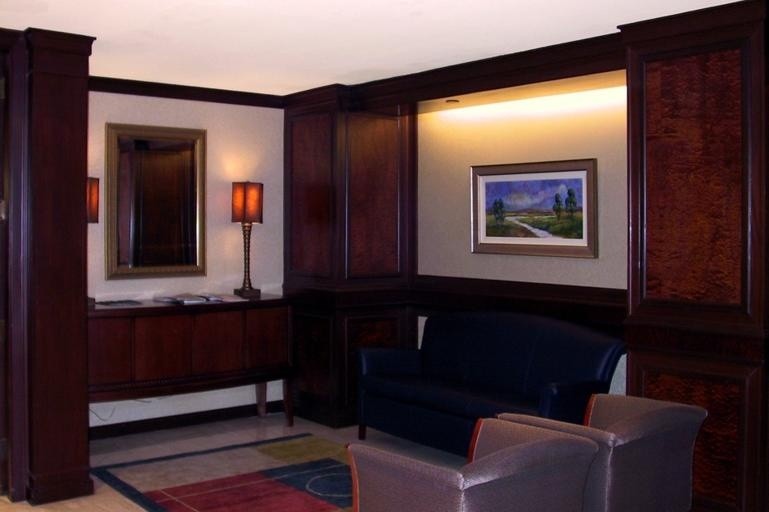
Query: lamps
xmin=88 ymin=177 xmax=100 ymax=224
xmin=230 ymin=182 xmax=265 ymax=300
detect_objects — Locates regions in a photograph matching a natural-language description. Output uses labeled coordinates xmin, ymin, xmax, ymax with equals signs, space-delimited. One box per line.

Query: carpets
xmin=91 ymin=433 xmax=355 ymax=511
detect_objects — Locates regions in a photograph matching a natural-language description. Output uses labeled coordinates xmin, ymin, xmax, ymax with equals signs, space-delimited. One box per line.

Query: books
xmin=95 ymin=291 xmax=225 ymax=305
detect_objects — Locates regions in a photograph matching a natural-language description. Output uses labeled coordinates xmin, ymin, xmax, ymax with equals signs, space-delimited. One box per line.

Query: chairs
xmin=345 ymin=415 xmax=599 ymax=512
xmin=496 ymin=392 xmax=707 ymax=511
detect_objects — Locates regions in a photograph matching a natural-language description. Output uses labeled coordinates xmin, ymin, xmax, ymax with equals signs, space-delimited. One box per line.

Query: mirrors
xmin=105 ymin=121 xmax=209 ymax=281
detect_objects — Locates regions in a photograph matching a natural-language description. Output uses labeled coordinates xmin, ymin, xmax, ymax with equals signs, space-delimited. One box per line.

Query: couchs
xmin=348 ymin=303 xmax=626 ymax=462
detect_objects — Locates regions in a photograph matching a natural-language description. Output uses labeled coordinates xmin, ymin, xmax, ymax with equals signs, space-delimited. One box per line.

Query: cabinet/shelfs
xmin=86 ymin=293 xmax=298 ymax=429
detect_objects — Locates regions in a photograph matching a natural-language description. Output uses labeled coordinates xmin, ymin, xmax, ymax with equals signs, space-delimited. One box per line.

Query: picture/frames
xmin=468 ymin=157 xmax=600 ymax=260
